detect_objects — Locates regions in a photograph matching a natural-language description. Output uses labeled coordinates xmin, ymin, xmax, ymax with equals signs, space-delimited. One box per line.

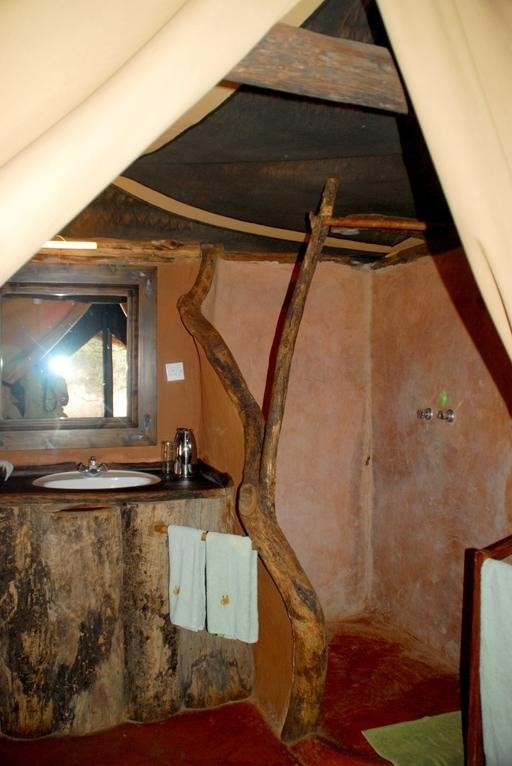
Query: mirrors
xmin=0 ymin=262 xmax=159 ymax=450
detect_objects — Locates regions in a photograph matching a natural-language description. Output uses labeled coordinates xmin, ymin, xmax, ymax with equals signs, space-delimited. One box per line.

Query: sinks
xmin=32 ymin=470 xmax=162 ymax=489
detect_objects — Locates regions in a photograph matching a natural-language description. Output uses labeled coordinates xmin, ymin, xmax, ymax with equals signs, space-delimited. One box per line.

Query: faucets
xmin=76 ymin=456 xmax=109 ymax=476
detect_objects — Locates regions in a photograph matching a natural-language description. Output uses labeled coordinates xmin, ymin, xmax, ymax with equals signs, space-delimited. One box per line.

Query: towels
xmin=167 ymin=523 xmax=260 ymax=645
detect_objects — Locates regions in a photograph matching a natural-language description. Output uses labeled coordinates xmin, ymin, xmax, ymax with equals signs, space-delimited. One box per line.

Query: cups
xmin=160 ymin=441 xmax=181 ymax=480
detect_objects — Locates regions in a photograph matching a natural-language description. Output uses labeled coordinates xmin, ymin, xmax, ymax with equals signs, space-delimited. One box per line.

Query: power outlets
xmin=166 ymin=361 xmax=185 ymax=382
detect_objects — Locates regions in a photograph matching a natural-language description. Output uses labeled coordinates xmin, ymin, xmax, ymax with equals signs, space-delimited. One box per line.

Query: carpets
xmin=360 ymin=710 xmax=463 ymax=765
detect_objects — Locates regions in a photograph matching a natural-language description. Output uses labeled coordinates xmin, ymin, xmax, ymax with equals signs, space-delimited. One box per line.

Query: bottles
xmin=173 ymin=427 xmax=199 ymax=478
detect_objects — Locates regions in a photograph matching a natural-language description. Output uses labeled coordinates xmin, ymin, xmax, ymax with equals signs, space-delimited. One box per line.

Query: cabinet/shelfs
xmin=467 ymin=535 xmax=512 ymax=766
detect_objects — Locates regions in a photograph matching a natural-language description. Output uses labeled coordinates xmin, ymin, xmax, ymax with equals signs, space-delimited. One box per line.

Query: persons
xmin=13 ymin=348 xmax=70 ymax=419
